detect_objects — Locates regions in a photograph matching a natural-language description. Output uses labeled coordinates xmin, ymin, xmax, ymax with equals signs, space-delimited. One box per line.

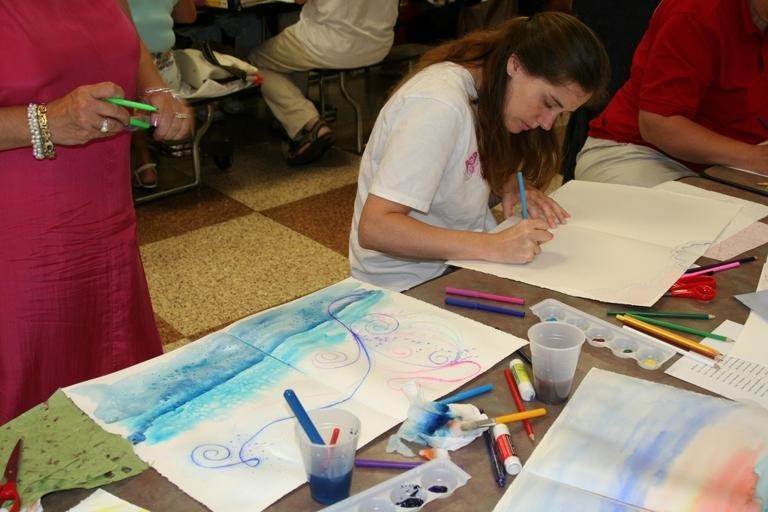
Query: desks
xmin=704 ymin=144 xmax=768 ymax=194
xmin=1 ymin=176 xmax=767 ymax=512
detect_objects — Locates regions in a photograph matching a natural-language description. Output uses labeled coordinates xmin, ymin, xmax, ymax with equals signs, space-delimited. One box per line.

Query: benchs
xmin=132 ymin=45 xmax=446 ymax=209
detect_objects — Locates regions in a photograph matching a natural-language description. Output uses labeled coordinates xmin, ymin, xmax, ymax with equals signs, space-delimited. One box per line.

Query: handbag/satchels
xmin=174 ymin=48 xmax=259 ymax=89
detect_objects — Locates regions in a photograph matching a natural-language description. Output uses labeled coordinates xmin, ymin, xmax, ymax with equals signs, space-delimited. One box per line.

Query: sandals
xmin=132 ymin=163 xmax=158 ymax=190
xmin=287 ymin=119 xmax=334 ymax=166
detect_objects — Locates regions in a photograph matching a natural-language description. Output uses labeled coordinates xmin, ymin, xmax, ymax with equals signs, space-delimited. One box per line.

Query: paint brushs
xmin=461 ymin=408 xmax=546 ymax=431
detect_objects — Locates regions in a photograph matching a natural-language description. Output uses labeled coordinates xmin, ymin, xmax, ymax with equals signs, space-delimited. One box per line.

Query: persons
xmin=127 ymin=0 xmax=182 ymax=189
xmin=348 ymin=12 xmax=611 ymax=294
xmin=400 ymin=0 xmax=574 ymax=35
xmin=246 ymin=0 xmax=399 ymax=165
xmin=0 ymin=0 xmax=193 ymax=429
xmin=575 ymin=0 xmax=768 ymax=188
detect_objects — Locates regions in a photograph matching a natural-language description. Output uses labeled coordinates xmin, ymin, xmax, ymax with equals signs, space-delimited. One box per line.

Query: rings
xmin=99 ymin=119 xmax=108 ymax=134
xmin=175 ymin=113 xmax=192 ymax=119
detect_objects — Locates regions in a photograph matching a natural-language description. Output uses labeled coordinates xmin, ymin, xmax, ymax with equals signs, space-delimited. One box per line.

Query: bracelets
xmin=143 ymin=88 xmax=172 ymax=93
xmin=26 ymin=103 xmax=44 ymax=161
xmin=37 ymin=104 xmax=55 ymax=160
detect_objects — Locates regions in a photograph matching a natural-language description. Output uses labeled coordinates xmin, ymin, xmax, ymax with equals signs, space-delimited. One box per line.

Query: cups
xmin=295 ymin=409 xmax=361 ymax=506
xmin=527 ymin=321 xmax=586 ymax=405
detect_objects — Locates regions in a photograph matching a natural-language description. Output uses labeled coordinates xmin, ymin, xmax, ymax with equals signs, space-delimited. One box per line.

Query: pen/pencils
xmin=329 ymin=428 xmax=340 ymax=445
xmin=484 ymin=429 xmax=505 ymax=487
xmin=756 ymin=182 xmax=768 ymax=186
xmin=608 ymin=310 xmax=735 ymax=370
xmin=354 ymin=459 xmax=463 ymax=470
xmin=517 ymin=172 xmax=528 ymax=219
xmin=101 ymin=97 xmax=160 ymax=112
xmin=445 ymin=288 xmax=525 ymax=317
xmin=284 ymin=389 xmax=325 ymax=445
xmin=437 ymin=384 xmax=493 ymax=405
xmin=504 ymin=368 xmax=535 ymax=441
xmin=130 ymin=118 xmax=150 ymax=129
xmin=683 ymin=256 xmax=757 ymax=277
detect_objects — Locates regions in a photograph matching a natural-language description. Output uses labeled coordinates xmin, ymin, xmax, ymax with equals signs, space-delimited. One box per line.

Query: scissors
xmin=0 ymin=438 xmax=23 ymax=512
xmin=664 ymin=275 xmax=716 ymax=300
